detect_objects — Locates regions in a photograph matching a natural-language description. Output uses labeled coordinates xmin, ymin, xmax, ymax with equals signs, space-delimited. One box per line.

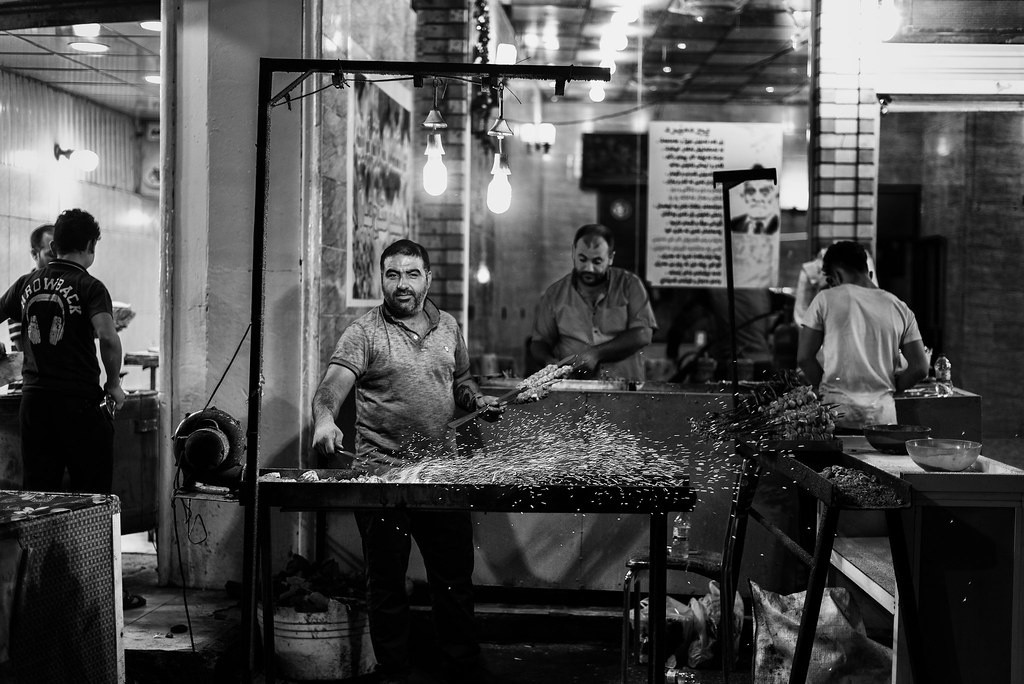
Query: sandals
xmin=123 ymin=590 xmax=147 ymax=609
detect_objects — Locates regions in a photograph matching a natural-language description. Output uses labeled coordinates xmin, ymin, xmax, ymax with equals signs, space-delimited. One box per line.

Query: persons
xmin=792 ymin=241 xmax=929 ymax=431
xmin=792 ymin=239 xmax=881 ymax=333
xmin=306 ymin=237 xmax=507 ymax=684
xmin=665 ymin=285 xmax=789 ymax=386
xmin=529 ymin=224 xmax=656 ymax=386
xmin=0 ymin=208 xmax=146 ymax=609
xmin=730 ymin=168 xmax=779 ymax=236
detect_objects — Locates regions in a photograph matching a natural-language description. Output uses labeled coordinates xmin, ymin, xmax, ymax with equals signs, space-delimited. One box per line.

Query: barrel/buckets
xmin=256 ymin=597 xmax=378 ymax=679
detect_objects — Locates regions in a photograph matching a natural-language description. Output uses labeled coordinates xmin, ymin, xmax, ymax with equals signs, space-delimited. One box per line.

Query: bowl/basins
xmin=862 ymin=425 xmax=932 ymax=455
xmin=905 ymin=439 xmax=982 ymax=472
xmin=904 ymin=388 xmax=926 ymax=397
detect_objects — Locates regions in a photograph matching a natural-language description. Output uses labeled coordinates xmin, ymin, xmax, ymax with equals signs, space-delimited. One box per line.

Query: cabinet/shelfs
xmin=815 ymin=435 xmax=1024 ymax=684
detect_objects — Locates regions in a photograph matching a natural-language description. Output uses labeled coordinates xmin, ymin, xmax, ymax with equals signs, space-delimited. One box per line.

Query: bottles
xmin=934 ymin=354 xmax=953 ymax=397
